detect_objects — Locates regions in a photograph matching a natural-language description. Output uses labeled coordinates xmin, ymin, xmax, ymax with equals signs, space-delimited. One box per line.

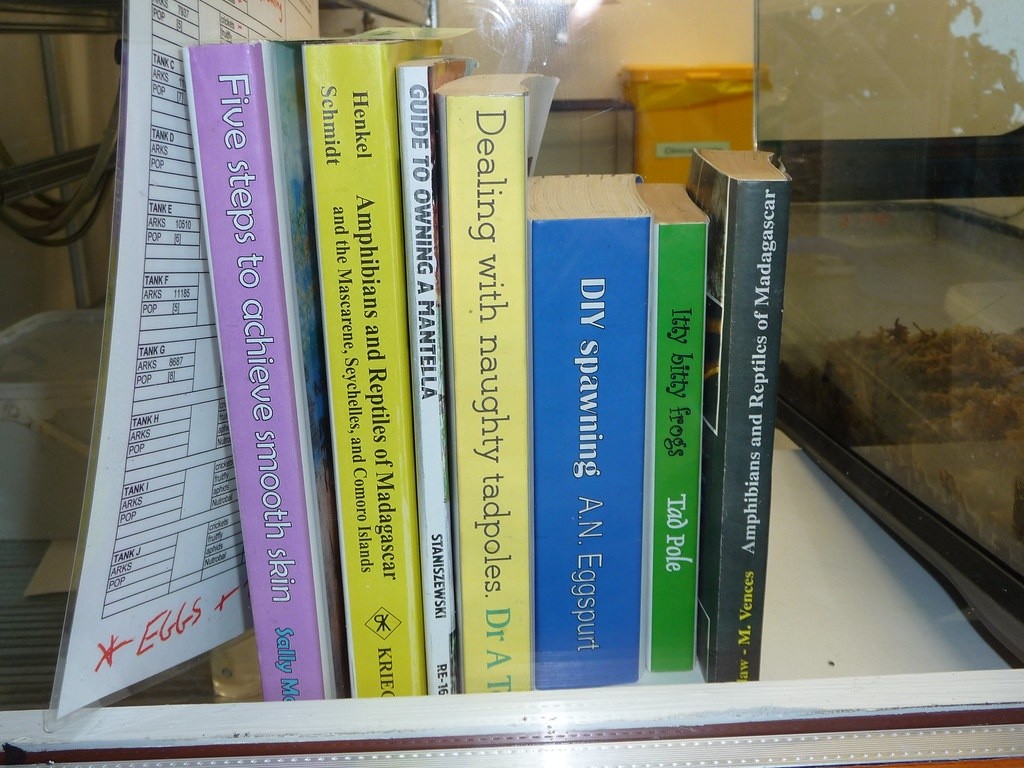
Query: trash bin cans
xmin=617 ymin=60 xmax=773 ymax=183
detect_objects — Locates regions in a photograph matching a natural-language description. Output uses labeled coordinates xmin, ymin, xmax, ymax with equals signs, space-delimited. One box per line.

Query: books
xmin=682 ymin=145 xmax=791 ymax=684
xmin=184 ymin=44 xmax=711 ymax=701
